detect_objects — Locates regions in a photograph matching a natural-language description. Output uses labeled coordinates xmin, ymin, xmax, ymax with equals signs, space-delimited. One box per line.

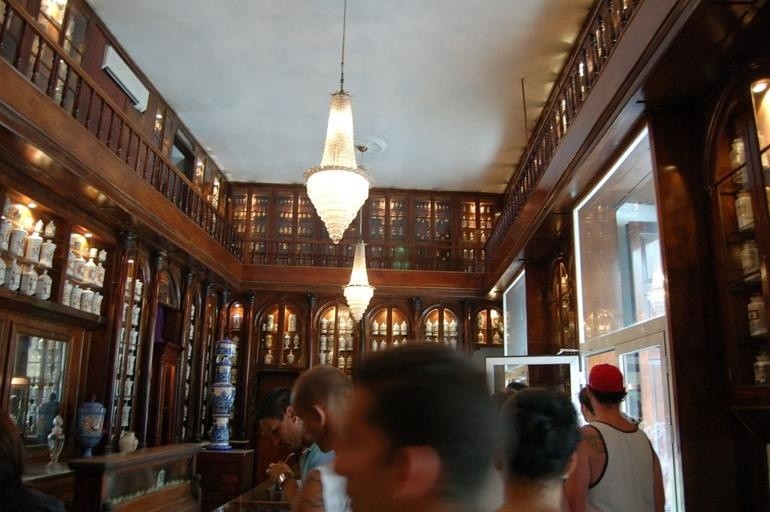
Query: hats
xmin=589 ymin=364 xmax=623 ymax=392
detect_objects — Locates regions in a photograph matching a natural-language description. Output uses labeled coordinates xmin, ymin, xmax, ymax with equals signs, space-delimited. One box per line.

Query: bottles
xmin=729 ymin=137 xmax=770 ymax=386
xmin=261 ymin=310 xmax=503 ymax=370
xmin=113 ymin=276 xmax=144 ymax=441
xmin=1 ymin=195 xmax=107 ymax=315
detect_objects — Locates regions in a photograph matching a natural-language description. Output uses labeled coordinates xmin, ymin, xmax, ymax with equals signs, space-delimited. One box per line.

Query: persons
xmin=579 ymin=387 xmax=596 ymax=423
xmin=0 ymin=408 xmax=66 ymax=511
xmin=253 ymin=343 xmax=529 ymax=511
xmin=564 ymin=362 xmax=668 ymax=512
xmin=491 ymin=387 xmax=582 ymax=511
